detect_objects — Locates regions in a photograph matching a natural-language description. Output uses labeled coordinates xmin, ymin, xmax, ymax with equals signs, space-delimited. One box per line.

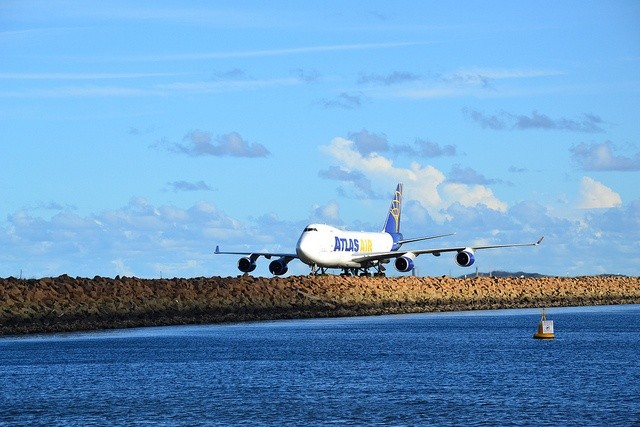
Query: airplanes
xmin=214 ymin=184 xmax=545 ymax=277
